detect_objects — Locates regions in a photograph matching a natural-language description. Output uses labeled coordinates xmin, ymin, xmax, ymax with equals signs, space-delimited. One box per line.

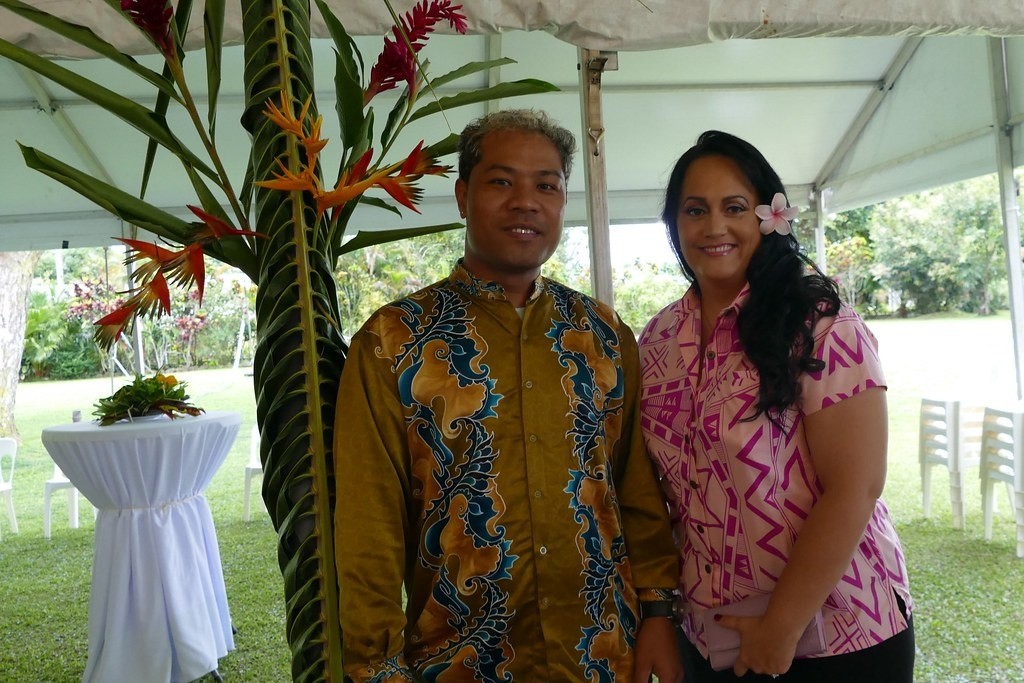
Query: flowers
xmin=92 ymin=365 xmax=208 ymax=426
xmin=755 ymin=192 xmax=800 ymax=234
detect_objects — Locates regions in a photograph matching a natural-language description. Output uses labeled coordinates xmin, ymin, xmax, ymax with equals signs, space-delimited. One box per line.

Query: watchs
xmin=638 ymin=596 xmax=683 ymax=619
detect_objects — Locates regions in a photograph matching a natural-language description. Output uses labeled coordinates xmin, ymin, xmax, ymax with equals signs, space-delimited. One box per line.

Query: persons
xmin=333 ymin=110 xmax=683 ymax=683
xmin=638 ymin=129 xmax=915 ymax=683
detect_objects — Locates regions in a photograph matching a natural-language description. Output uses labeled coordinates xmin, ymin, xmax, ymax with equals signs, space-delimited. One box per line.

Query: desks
xmin=42 ymin=409 xmax=245 ymax=683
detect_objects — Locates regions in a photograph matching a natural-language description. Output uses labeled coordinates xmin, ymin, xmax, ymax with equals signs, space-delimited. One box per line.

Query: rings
xmin=772 ymin=673 xmax=779 ymax=679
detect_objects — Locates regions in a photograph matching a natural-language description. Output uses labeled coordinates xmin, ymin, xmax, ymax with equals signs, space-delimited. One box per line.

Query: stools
xmin=919 ymin=399 xmax=1024 ymax=557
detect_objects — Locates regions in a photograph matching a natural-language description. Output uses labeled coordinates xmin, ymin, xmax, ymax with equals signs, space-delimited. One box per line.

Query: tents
xmin=0 ymin=0 xmax=1024 ymax=455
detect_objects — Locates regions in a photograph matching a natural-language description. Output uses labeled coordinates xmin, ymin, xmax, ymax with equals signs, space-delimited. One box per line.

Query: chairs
xmin=242 ymin=424 xmax=268 ymax=522
xmin=44 ymin=465 xmax=100 ymax=539
xmin=1 ymin=438 xmax=18 ymax=532
